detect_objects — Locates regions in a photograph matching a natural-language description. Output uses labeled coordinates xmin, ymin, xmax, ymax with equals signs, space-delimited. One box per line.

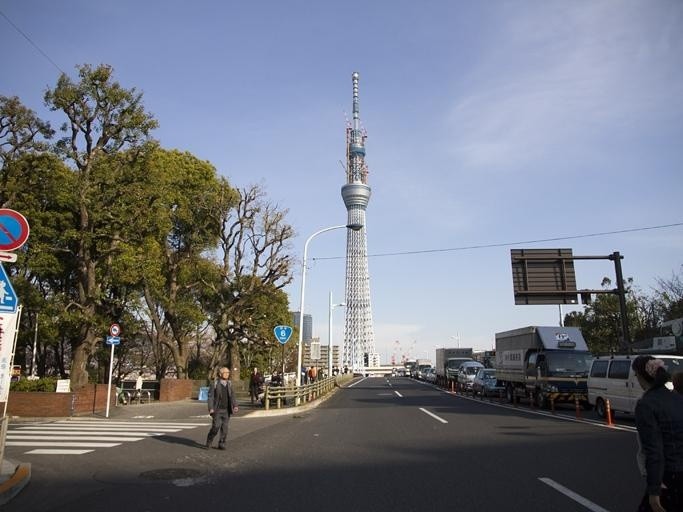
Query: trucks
xmin=495 ymin=324 xmax=594 ymax=411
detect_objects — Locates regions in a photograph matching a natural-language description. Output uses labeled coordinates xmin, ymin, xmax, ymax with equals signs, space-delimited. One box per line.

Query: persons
xmin=205 ymin=367 xmax=238 ymax=451
xmin=249 ymin=364 xmax=349 ymax=405
xmin=116 ymin=372 xmax=128 ymax=405
xmin=632 ymin=355 xmax=683 ymax=512
xmin=130 ymin=369 xmax=144 ymax=405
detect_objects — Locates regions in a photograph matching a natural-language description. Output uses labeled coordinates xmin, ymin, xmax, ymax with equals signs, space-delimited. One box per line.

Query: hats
xmin=219 ymin=367 xmax=230 ymax=376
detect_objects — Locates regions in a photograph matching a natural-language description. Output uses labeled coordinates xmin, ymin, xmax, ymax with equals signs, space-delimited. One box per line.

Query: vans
xmin=587 ymin=353 xmax=683 ymax=419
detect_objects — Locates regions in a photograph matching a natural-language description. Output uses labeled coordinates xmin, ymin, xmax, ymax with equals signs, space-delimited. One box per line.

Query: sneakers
xmin=219 ymin=443 xmax=225 ymax=450
xmin=206 ymin=441 xmax=212 ymax=447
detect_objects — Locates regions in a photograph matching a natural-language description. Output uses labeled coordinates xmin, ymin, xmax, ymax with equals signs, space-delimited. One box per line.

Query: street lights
xmin=294 ymin=222 xmax=365 ymax=407
xmin=328 ymin=290 xmax=349 ymax=378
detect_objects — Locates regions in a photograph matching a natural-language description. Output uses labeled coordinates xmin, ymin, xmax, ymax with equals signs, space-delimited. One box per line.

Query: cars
xmin=402 ymin=339 xmax=501 ymax=397
